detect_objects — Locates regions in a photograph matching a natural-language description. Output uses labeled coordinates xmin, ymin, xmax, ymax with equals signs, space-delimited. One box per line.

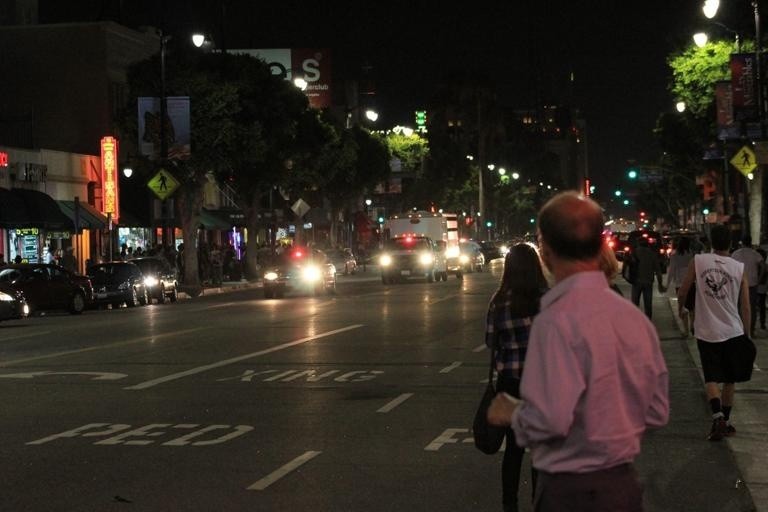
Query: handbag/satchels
xmin=472 ymin=378 xmax=507 ymax=455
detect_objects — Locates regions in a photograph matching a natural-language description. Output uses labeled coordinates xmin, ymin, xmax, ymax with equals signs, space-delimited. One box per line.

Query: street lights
xmin=341 ymin=98 xmax=386 ymax=249
xmin=699 ymin=0 xmax=767 ymax=246
xmin=688 ymin=19 xmax=751 ymax=243
xmin=156 ymin=23 xmax=215 ymax=261
xmin=268 ymin=65 xmax=311 ymax=271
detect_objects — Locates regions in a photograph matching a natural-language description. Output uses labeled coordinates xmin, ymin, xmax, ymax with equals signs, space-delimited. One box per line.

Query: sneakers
xmin=704 ymin=416 xmax=736 ymax=441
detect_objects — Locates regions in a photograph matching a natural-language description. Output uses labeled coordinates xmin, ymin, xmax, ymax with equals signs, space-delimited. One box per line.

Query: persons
xmin=0 ymin=240 xmax=292 ymax=293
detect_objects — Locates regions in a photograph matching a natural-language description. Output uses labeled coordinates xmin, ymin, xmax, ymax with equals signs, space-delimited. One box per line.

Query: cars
xmin=259 ymin=248 xmax=340 ymax=302
xmin=126 ymin=255 xmax=182 ymax=306
xmin=0 ymin=278 xmax=32 ymax=321
xmin=86 ymin=259 xmax=150 ymax=309
xmin=319 ymin=247 xmax=358 ymax=277
xmin=0 ymin=261 xmax=95 ymax=318
